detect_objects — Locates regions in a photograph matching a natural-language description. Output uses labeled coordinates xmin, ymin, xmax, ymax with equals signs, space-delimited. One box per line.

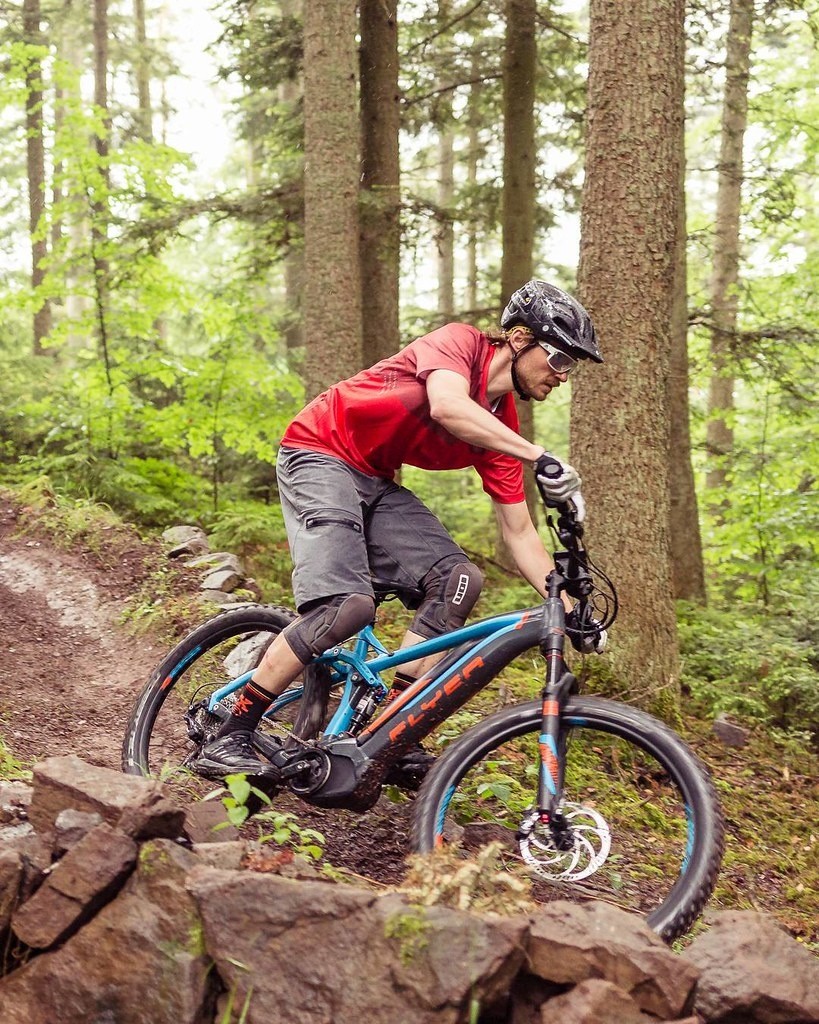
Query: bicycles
xmin=118 ymin=456 xmax=728 ymax=949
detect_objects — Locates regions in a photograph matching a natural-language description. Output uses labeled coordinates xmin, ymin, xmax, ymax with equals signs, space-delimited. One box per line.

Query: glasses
xmin=539 ymin=340 xmax=579 ymax=374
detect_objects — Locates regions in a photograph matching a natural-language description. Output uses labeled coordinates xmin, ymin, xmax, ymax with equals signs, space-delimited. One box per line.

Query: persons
xmin=190 ymin=280 xmax=608 ymax=793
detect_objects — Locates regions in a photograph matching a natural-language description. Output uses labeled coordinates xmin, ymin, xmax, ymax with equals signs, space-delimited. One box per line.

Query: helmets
xmin=501 ymin=279 xmax=605 ymax=364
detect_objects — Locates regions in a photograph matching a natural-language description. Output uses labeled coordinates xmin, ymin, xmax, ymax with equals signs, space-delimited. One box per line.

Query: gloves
xmin=570 ymin=618 xmax=608 ymax=652
xmin=537 ymin=451 xmax=587 ymax=522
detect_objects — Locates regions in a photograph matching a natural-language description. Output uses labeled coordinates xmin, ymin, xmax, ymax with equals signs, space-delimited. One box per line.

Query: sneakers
xmin=388 ymin=743 xmax=437 ymax=791
xmin=192 ymin=730 xmax=265 ymax=774
